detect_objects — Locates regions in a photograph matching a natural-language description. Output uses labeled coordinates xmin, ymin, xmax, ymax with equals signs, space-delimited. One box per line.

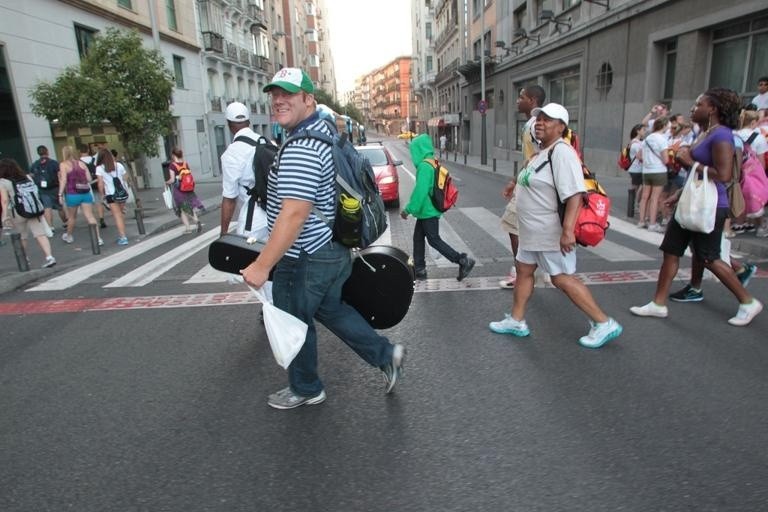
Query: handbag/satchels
xmin=618 ymin=144 xmax=631 ymax=171
xmin=548 ymin=142 xmax=610 ymax=247
xmin=163 ymin=191 xmax=173 ymax=210
xmin=73 ymin=182 xmax=90 ymax=193
xmin=724 ymin=143 xmax=768 ymax=218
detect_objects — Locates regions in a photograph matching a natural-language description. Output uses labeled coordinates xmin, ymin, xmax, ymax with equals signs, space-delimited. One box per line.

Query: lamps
xmin=481 ymin=1 xmax=611 ymax=62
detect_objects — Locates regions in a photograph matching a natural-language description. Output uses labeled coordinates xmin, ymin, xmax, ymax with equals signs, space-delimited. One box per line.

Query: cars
xmin=351 ymin=141 xmax=406 ymax=210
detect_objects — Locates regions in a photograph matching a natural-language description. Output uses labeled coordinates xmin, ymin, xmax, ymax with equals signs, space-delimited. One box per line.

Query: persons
xmin=164 ymin=149 xmax=204 ymax=235
xmin=499 ymin=85 xmax=546 ymax=290
xmin=627 ymin=77 xmax=768 ymax=326
xmin=239 ymin=66 xmax=404 ymax=409
xmin=439 ymin=133 xmax=446 ymax=154
xmin=218 ymin=102 xmax=274 ymax=326
xmin=489 ymin=103 xmax=624 ymax=348
xmin=401 ymin=133 xmax=476 ymax=283
xmin=0 ymin=145 xmax=136 ymax=268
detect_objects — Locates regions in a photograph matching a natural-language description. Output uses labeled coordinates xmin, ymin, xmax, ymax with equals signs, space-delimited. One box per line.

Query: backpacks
xmin=0 ymin=176 xmax=45 ymax=218
xmin=172 ymin=162 xmax=194 ymax=192
xmin=276 ymin=120 xmax=387 ymax=250
xmin=34 ymin=159 xmax=59 ymax=191
xmin=100 ymin=162 xmax=128 ymax=204
xmin=79 ymin=158 xmax=98 ymax=190
xmin=423 ymin=157 xmax=458 ymax=212
xmin=234 ymin=136 xmax=278 ymax=211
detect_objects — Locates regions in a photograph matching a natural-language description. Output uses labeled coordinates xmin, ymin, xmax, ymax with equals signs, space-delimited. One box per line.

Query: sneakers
xmin=117 ymin=237 xmax=128 ymax=245
xmin=725 ymin=222 xmax=768 ymax=238
xmin=489 ymin=313 xmax=530 ymax=337
xmin=728 ymin=297 xmax=763 ymax=326
xmin=629 ymin=301 xmax=668 ymax=318
xmin=40 ymin=258 xmax=57 ymax=268
xmin=62 ymin=233 xmax=74 ymax=243
xmin=265 ymin=386 xmax=326 ymax=410
xmin=379 ymin=344 xmax=407 ymax=393
xmin=579 ymin=317 xmax=623 ymax=348
xmin=638 ymin=217 xmax=670 ymax=232
xmin=499 ymin=272 xmax=517 ymax=289
xmin=98 ymin=238 xmax=105 ymax=246
xmin=669 ymin=284 xmax=704 ymax=302
xmin=736 ymin=262 xmax=758 ymax=288
xmin=457 ymin=253 xmax=475 ymax=281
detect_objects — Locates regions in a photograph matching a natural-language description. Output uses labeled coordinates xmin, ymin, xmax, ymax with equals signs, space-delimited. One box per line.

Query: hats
xmin=531 ymin=102 xmax=569 ymax=127
xmin=262 ymin=68 xmax=315 ymax=93
xmin=225 ymin=102 xmax=250 ymax=123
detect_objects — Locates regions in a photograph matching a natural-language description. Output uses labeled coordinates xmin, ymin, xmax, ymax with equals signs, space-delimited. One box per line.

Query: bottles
xmin=339 ymin=193 xmax=360 ymax=246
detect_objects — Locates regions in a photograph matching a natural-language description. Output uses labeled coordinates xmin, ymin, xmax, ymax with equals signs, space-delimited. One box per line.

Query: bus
xmin=270 ymin=102 xmax=366 ymax=147
